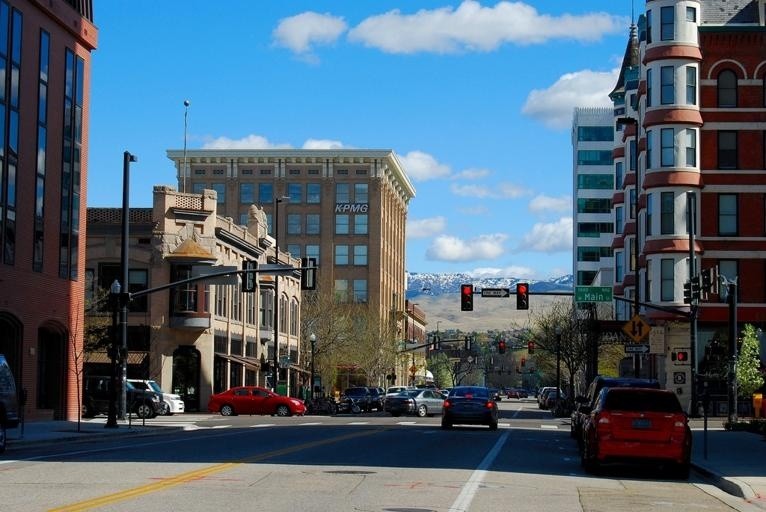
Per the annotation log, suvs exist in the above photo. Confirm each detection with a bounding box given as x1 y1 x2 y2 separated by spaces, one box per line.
82 375 185 419
0 353 19 453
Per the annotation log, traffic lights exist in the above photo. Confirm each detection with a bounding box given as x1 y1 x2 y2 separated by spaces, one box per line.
528 340 534 354
671 352 688 362
499 340 505 354
461 284 473 311
498 358 534 376
684 269 709 304
517 283 528 310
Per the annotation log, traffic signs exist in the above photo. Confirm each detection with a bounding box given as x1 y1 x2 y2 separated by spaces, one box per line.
574 286 613 302
625 344 650 354
482 288 510 297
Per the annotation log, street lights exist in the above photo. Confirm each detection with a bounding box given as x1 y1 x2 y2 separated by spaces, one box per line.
104 279 121 428
309 333 316 400
618 116 641 314
555 325 563 402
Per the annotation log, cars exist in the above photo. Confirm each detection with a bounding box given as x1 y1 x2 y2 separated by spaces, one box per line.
305 385 453 417
571 376 692 470
442 386 499 431
532 386 568 410
208 386 307 416
505 388 528 399
